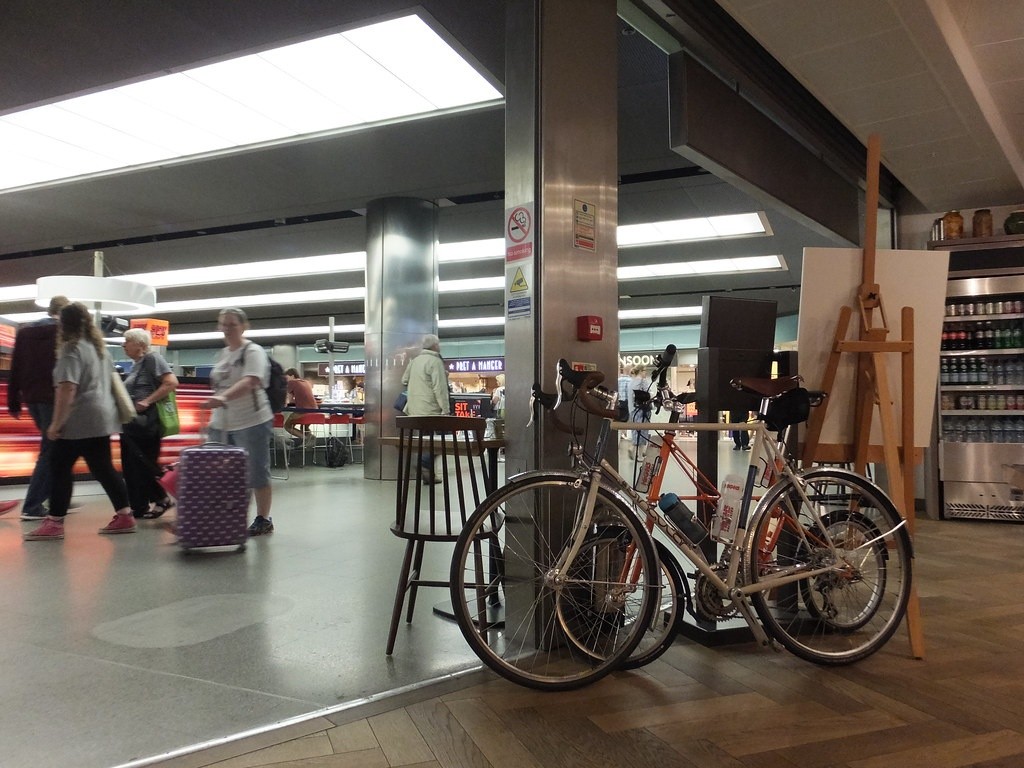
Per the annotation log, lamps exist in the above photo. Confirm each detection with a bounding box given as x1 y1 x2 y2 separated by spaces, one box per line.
313 339 349 353
99 314 129 334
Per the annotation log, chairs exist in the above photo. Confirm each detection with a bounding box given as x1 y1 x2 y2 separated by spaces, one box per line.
385 414 505 655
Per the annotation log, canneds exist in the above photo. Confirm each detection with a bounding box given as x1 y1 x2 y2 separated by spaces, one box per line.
945 301 1023 315
941 394 1024 410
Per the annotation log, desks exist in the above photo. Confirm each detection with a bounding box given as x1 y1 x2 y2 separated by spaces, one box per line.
377 434 505 628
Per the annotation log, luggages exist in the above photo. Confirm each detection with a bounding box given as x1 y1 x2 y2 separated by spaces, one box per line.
118 422 180 500
177 402 250 555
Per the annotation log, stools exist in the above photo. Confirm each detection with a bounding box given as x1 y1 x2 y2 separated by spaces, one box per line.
268 412 366 479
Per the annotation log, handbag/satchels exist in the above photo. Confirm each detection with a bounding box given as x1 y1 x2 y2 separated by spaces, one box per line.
111 371 137 425
394 390 407 410
155 391 180 437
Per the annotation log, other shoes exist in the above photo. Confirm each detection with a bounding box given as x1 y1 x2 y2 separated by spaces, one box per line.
637 455 645 462
742 444 751 450
306 435 316 451
293 441 308 450
0 500 19 515
423 475 442 485
628 447 635 460
733 443 741 450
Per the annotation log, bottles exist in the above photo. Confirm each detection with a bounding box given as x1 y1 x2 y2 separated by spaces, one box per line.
634 434 663 493
940 320 1024 444
658 493 706 544
713 473 744 541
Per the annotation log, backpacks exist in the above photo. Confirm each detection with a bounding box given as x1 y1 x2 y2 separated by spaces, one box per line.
325 436 349 468
240 341 287 413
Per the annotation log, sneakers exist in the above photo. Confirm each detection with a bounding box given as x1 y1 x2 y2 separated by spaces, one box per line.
98 513 137 534
20 506 49 520
22 518 65 540
247 516 274 537
67 504 82 513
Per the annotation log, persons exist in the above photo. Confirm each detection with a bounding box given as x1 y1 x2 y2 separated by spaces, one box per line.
284 368 317 449
24 303 137 541
492 373 505 454
208 308 274 536
619 362 650 462
729 411 751 450
402 335 451 484
121 329 178 519
6 296 82 519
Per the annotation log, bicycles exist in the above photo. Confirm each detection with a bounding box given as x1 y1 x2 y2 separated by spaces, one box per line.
550 344 890 670
449 358 916 692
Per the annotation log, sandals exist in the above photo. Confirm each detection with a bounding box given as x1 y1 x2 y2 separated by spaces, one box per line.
142 498 173 520
113 509 140 520
